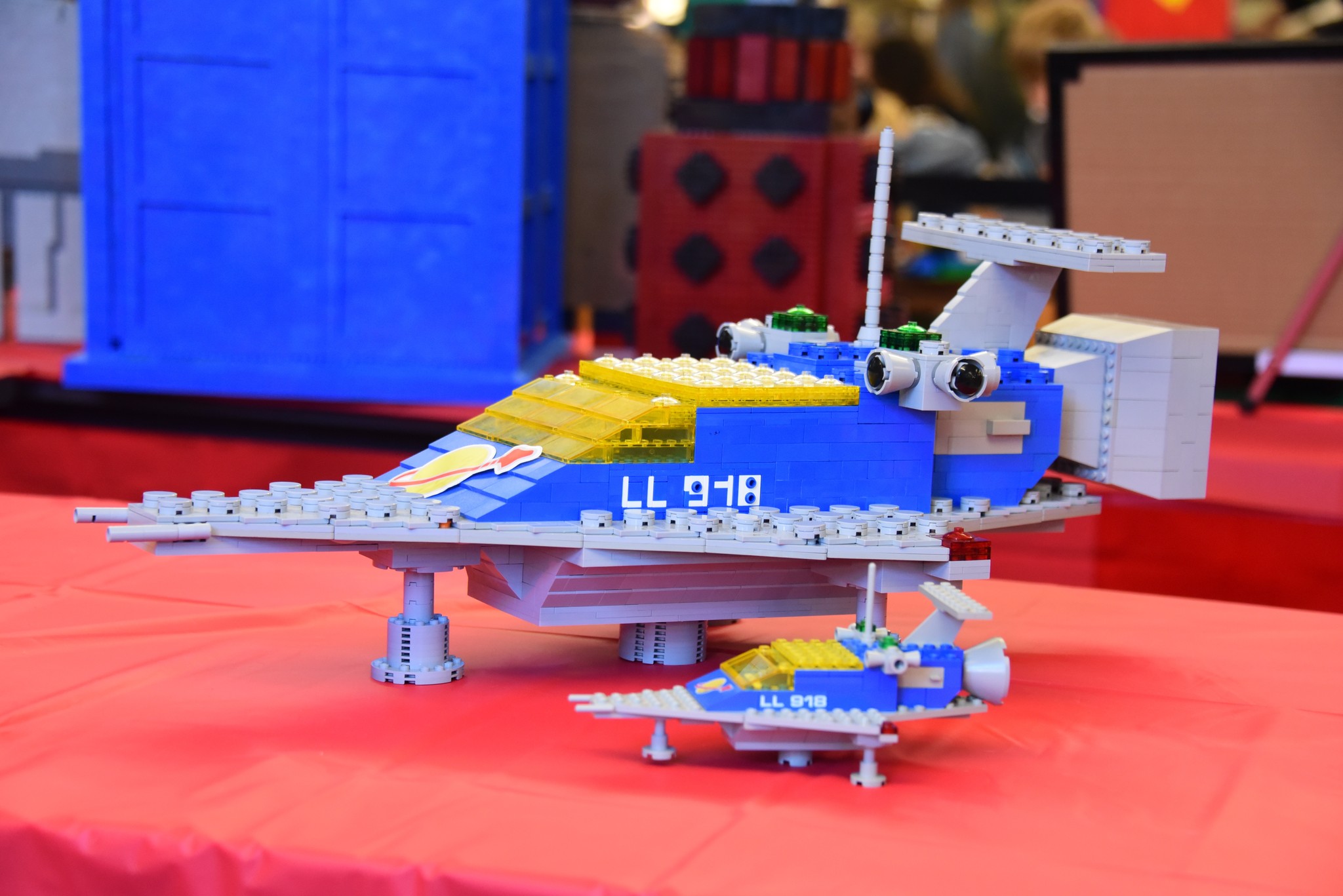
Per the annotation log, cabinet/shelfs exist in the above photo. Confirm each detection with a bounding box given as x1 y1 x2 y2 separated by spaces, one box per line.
64 0 567 407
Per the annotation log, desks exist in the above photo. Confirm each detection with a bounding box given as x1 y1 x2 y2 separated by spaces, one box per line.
0 493 1343 896
0 287 1343 616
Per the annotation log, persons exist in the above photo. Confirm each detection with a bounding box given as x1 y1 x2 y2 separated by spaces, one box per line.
994 0 1113 173
852 31 982 174
